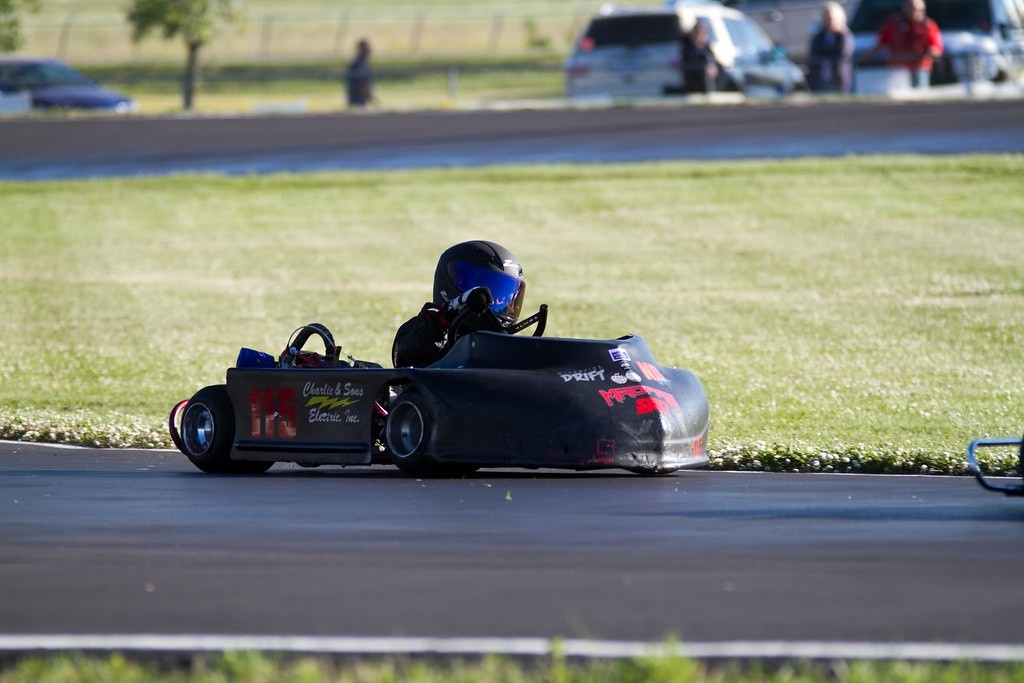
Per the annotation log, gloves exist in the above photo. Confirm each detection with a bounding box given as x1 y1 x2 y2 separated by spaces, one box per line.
438 286 494 326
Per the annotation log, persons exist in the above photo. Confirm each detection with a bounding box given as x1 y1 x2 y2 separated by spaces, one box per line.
684 25 717 96
808 2 854 94
883 0 943 88
392 240 526 369
343 39 374 111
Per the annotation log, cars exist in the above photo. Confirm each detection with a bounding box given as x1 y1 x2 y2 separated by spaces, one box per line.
810 0 1024 95
565 0 811 99
0 58 132 115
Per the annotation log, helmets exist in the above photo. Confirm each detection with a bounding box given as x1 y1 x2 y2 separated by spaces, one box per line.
432 239 524 327
